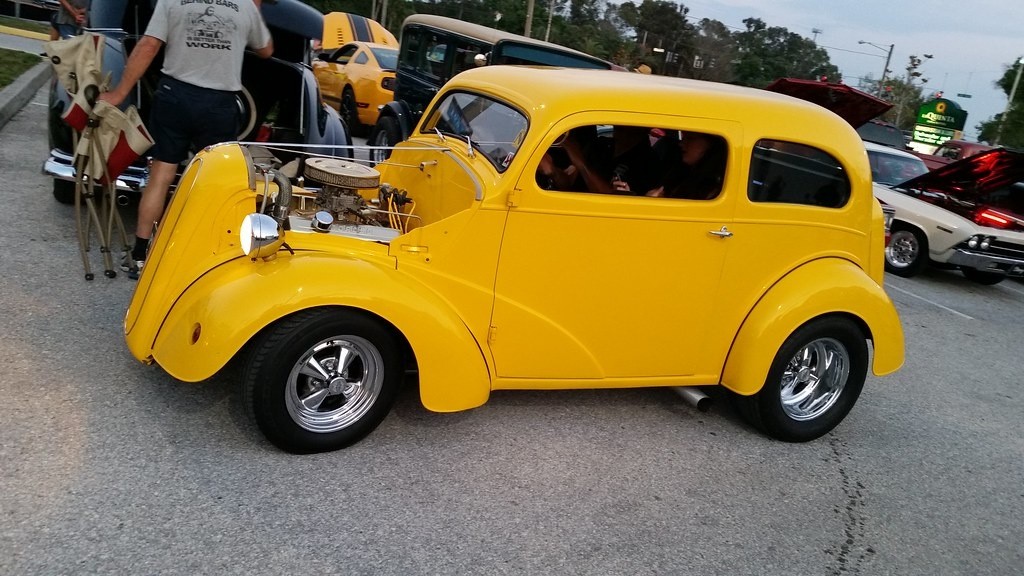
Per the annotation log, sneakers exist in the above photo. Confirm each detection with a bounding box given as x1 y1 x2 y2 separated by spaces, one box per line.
121 254 145 275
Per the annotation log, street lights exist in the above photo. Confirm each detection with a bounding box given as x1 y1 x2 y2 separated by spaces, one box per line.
858 39 894 99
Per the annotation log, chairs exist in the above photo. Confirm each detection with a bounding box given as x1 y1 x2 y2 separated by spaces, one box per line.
45 35 156 281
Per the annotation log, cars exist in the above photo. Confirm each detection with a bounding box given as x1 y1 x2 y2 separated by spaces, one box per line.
766 75 1024 285
41 5 628 206
121 64 904 456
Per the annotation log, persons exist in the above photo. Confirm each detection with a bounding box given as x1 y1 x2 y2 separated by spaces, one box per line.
95 0 273 273
541 124 719 199
635 64 652 74
40 0 89 57
